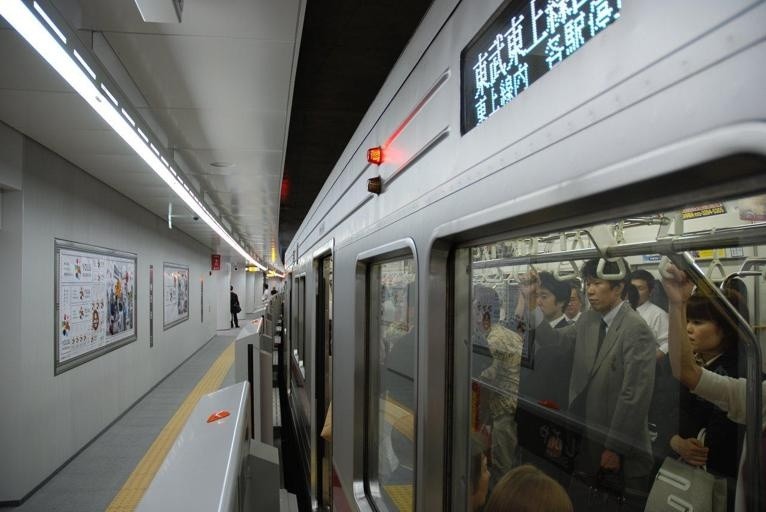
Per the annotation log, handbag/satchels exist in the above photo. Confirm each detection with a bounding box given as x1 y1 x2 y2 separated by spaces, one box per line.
644 457 727 512
234 304 241 313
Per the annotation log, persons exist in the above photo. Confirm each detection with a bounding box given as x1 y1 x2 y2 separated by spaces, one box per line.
230 285 241 328
261 283 269 301
271 286 278 299
379 255 765 512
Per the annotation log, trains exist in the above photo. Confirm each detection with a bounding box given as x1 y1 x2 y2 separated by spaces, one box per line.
275 2 766 512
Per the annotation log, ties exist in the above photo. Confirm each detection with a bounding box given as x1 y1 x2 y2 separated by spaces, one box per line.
594 319 607 365
